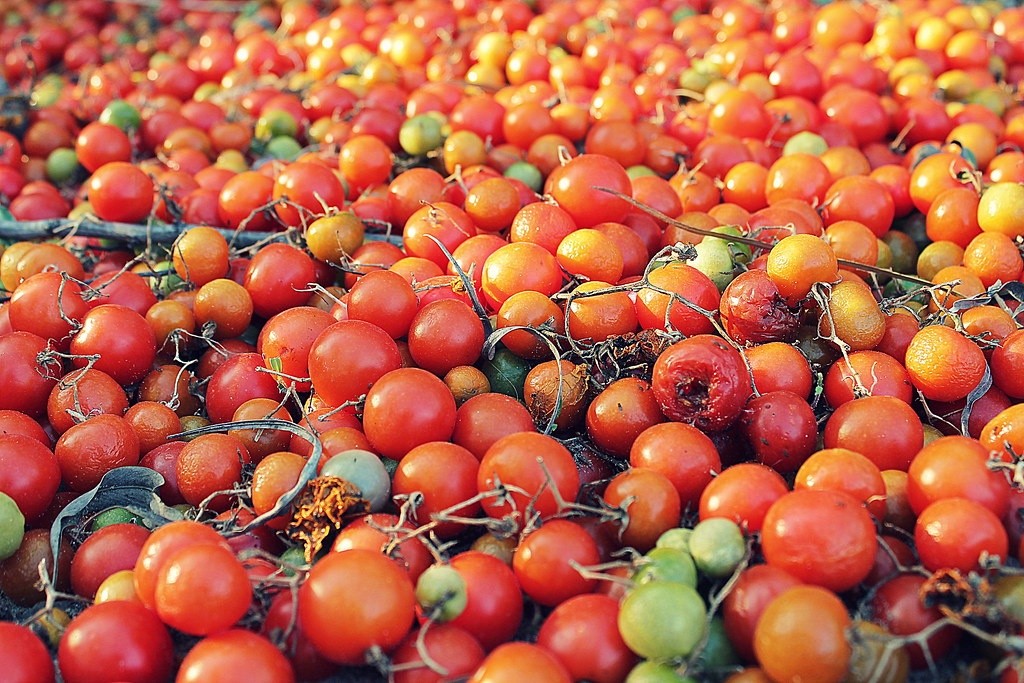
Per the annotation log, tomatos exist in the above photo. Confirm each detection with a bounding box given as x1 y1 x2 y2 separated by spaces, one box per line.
0 0 1024 683
